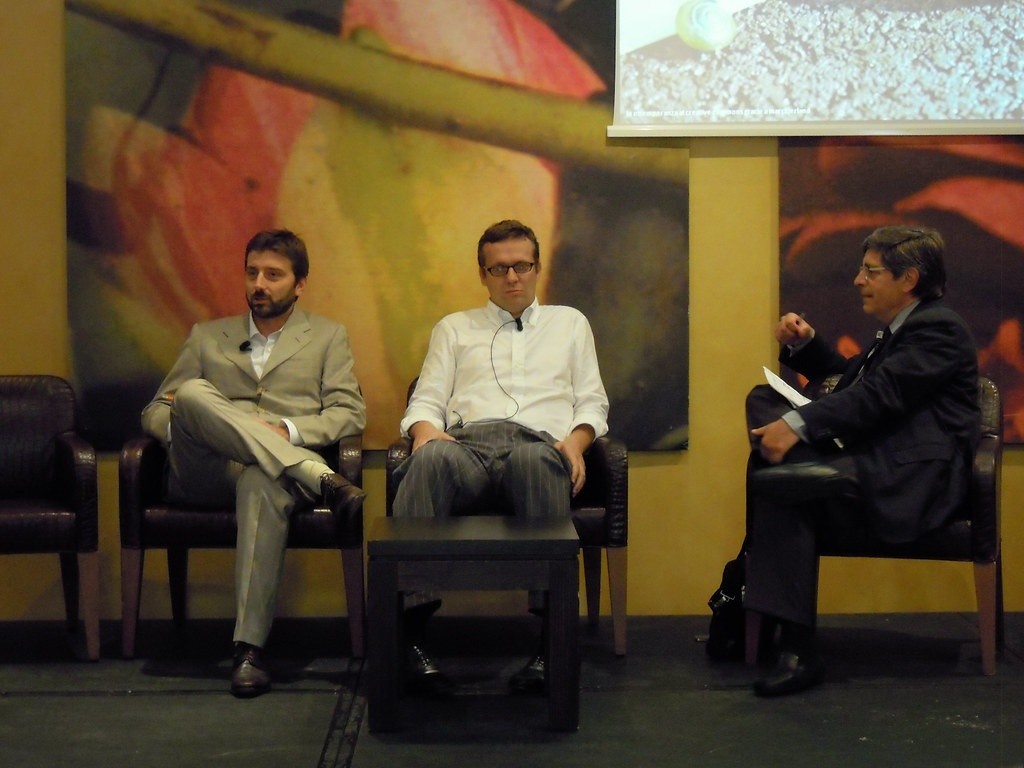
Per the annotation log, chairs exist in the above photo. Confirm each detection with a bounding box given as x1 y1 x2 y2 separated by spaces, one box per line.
0 375 100 663
122 363 364 663
386 373 629 656
747 372 1002 680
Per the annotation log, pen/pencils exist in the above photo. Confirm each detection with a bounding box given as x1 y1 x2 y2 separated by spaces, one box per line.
795 311 806 325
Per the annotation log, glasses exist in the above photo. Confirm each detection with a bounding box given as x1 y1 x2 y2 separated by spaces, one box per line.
858 265 889 282
482 261 537 277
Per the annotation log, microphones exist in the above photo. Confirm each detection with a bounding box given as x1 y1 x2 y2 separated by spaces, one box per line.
239 340 254 353
514 316 523 332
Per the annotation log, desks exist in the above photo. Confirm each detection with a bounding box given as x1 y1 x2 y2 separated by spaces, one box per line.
361 513 582 735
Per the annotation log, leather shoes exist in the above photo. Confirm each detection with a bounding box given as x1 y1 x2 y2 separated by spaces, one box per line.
319 472 365 523
752 647 825 696
755 463 860 495
231 641 272 699
399 641 438 682
509 645 550 690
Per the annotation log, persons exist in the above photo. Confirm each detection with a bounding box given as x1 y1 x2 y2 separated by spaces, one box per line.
142 227 366 694
393 219 610 693
750 227 982 701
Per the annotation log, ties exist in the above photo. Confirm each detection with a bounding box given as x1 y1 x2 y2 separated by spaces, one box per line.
858 325 892 376
